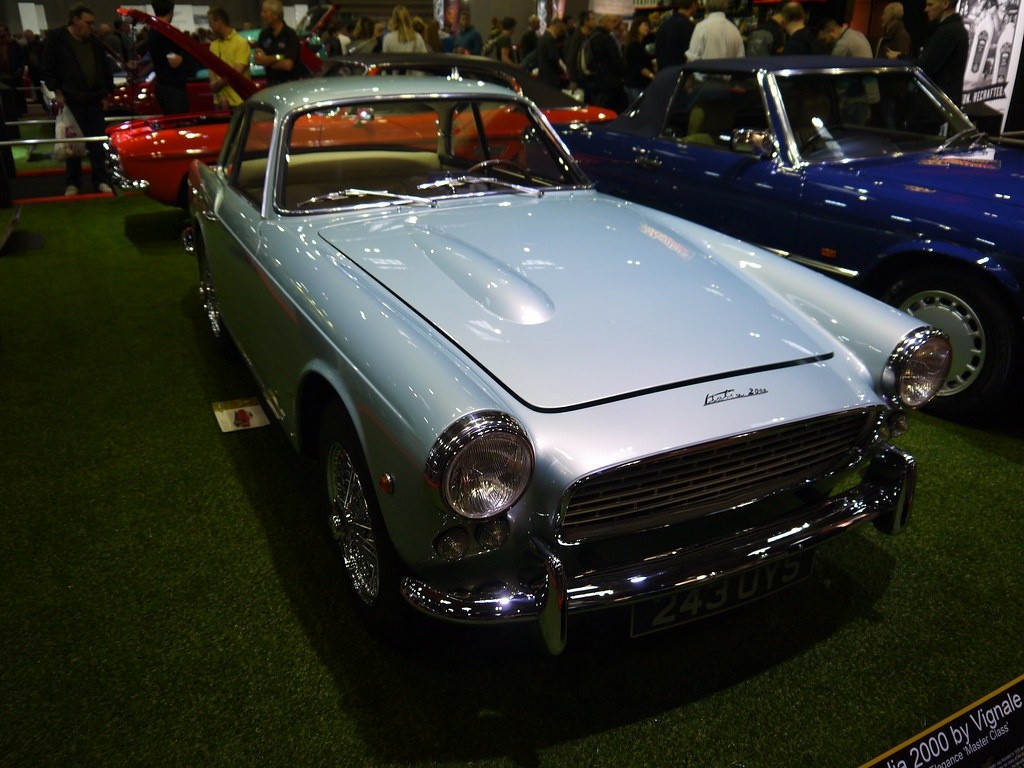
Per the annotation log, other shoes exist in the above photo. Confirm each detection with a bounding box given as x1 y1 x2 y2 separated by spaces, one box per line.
98 181 112 193
64 184 78 196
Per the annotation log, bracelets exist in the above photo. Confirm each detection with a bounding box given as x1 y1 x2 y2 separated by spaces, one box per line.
650 72 653 79
276 54 280 62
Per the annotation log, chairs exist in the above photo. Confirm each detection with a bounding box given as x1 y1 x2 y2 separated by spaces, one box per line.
789 95 846 158
681 98 737 152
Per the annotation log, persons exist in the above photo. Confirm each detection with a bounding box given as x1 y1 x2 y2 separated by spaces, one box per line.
1 0 968 206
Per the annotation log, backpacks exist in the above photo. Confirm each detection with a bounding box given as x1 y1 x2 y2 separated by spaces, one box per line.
576 32 605 86
747 28 773 57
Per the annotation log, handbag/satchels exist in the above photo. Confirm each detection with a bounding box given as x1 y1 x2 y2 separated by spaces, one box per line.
561 87 584 104
53 103 87 160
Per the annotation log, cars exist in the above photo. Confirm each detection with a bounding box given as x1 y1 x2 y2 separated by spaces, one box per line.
523 53 1024 426
100 51 618 226
187 75 951 641
100 0 337 124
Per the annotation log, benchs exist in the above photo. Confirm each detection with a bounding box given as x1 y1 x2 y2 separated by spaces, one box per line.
234 151 448 213
